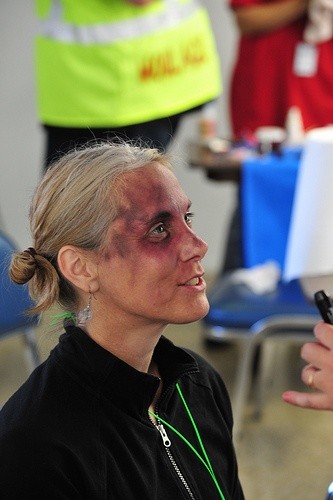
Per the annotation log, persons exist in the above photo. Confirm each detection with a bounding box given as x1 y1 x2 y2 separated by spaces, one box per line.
0 144 247 500
33 1 221 176
282 317 333 499
204 0 333 408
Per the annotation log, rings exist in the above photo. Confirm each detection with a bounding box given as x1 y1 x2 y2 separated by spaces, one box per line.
307 368 315 388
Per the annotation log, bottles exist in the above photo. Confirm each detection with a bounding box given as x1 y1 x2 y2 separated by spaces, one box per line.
293 14 319 77
200 99 219 148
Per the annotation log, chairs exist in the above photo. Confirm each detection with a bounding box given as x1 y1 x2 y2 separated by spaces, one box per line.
200 147 333 438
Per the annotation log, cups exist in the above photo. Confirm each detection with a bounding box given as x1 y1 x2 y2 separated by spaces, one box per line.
256 126 287 157
